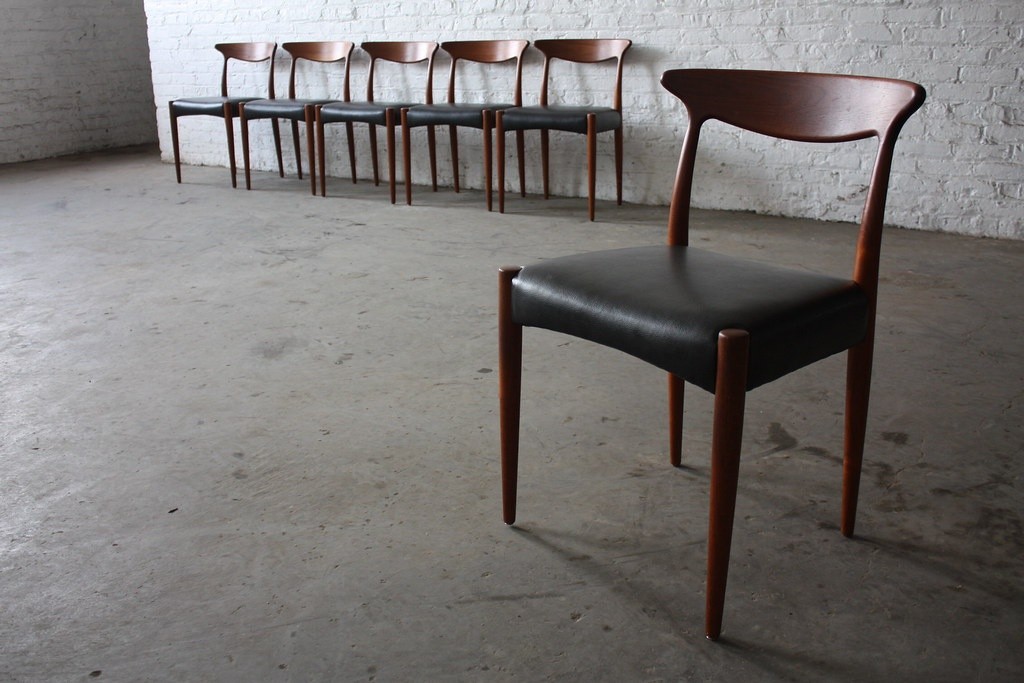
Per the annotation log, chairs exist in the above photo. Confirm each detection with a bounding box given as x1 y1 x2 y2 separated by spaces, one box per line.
497 67 927 641
238 41 357 196
168 42 284 189
314 42 438 205
400 40 530 211
496 38 632 222
